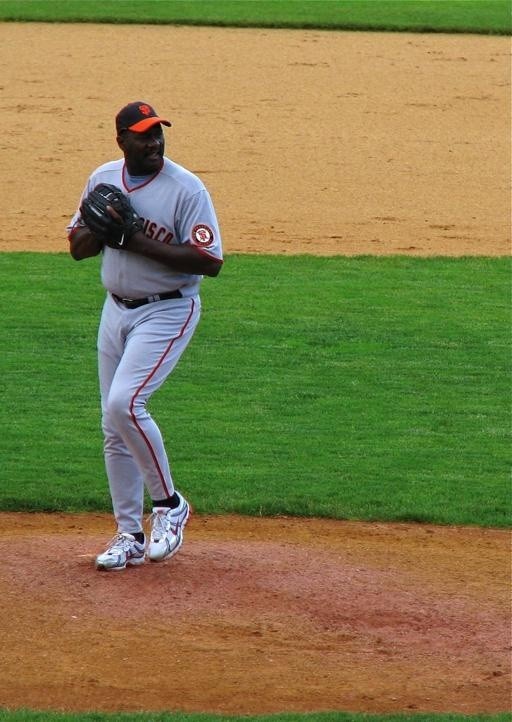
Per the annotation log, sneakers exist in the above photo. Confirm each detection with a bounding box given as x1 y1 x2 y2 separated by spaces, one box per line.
95 532 146 572
146 490 193 562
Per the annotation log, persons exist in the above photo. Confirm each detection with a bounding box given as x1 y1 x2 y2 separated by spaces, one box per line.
67 101 224 573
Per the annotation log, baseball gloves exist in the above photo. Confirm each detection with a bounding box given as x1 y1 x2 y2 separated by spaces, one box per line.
80 183 144 251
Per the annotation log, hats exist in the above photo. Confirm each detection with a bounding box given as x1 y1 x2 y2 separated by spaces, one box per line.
116 102 171 136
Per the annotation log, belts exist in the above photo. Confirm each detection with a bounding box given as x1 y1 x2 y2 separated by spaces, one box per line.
112 289 182 309
